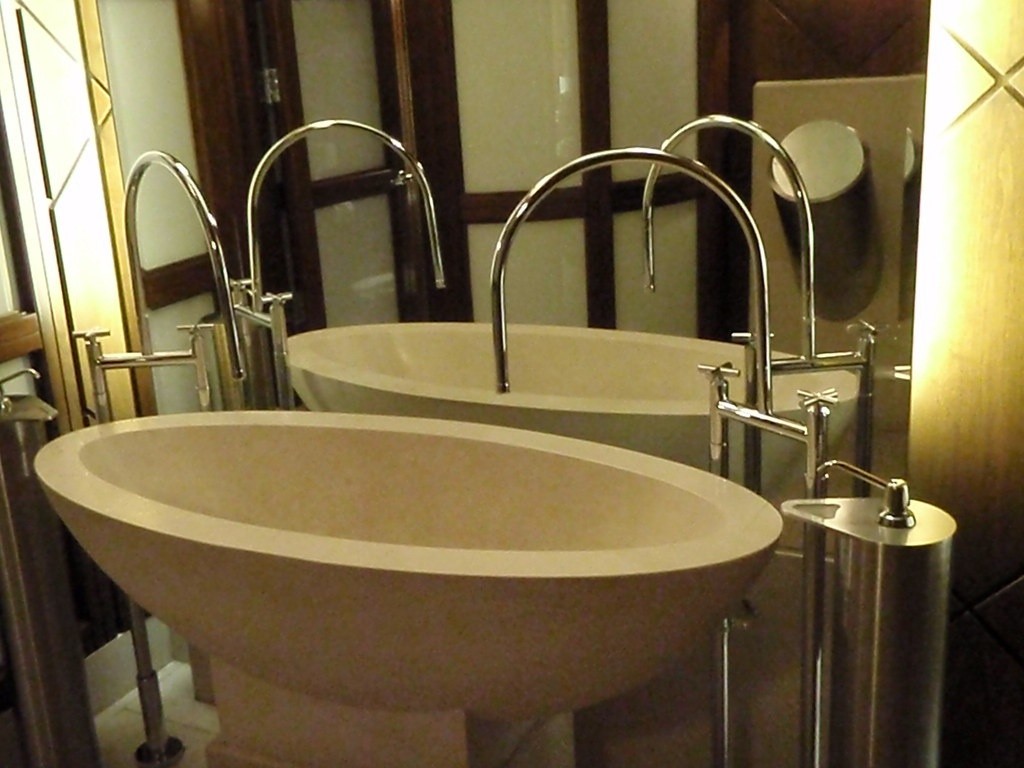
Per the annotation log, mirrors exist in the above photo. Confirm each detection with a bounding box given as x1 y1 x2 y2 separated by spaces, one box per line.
93 2 931 707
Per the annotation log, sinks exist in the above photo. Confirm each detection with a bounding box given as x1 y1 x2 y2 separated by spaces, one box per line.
276 321 859 491
32 410 785 711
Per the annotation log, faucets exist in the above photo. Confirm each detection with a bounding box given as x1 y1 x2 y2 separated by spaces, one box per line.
122 149 245 382
641 120 817 355
247 120 446 309
488 148 772 414
